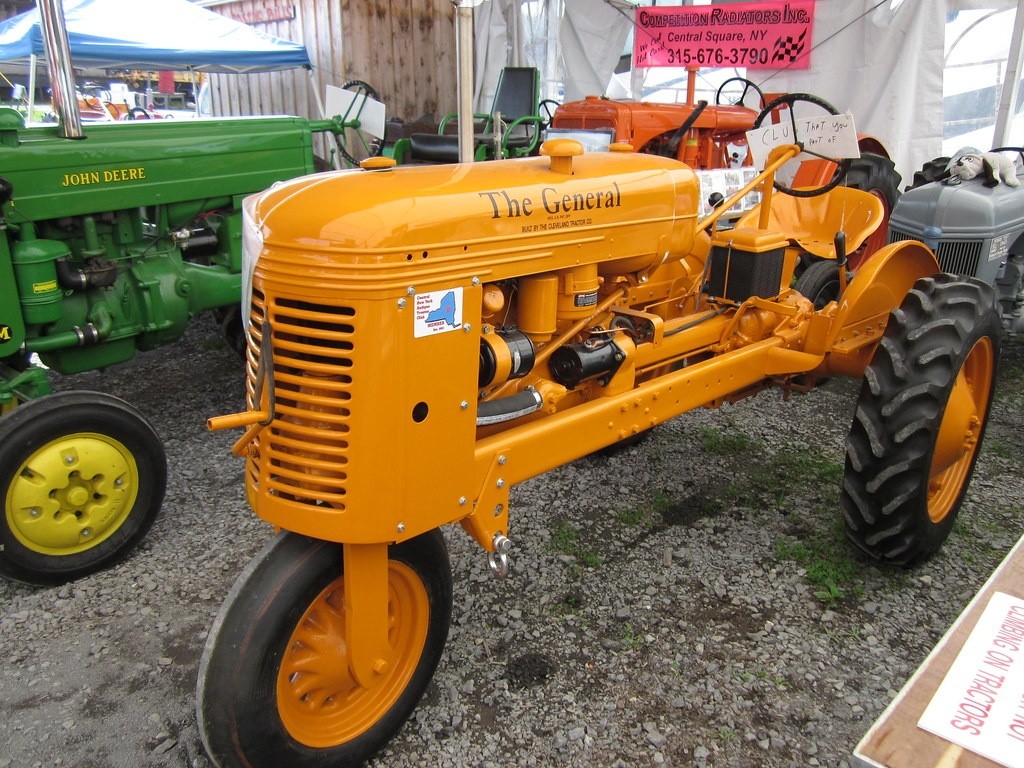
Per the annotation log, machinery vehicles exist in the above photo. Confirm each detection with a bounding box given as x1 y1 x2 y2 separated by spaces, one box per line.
0 0 551 591
179 71 1003 768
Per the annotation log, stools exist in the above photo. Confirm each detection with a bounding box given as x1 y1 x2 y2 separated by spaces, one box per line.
733 184 885 262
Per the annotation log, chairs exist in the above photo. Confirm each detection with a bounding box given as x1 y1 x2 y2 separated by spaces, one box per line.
409 63 540 166
